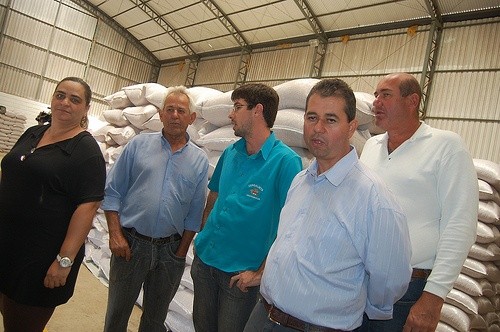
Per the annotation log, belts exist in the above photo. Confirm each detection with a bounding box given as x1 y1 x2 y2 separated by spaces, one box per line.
412 270 431 278
262 298 335 332
123 227 182 244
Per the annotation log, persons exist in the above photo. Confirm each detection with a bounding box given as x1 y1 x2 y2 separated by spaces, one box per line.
189 83 303 332
99 85 209 332
243 78 414 332
358 71 479 332
0 76 107 332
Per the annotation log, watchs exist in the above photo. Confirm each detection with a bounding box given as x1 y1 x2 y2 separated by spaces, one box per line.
57 254 73 268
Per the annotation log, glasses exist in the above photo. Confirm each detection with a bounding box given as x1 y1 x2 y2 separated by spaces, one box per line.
233 104 254 112
